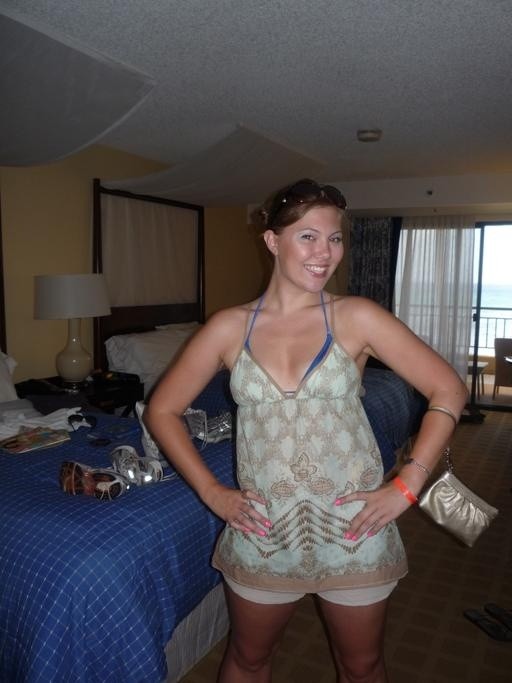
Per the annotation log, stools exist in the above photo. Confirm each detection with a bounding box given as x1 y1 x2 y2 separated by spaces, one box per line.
465 359 492 401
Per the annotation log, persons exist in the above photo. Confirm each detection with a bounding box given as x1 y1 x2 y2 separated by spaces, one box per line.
141 178 470 683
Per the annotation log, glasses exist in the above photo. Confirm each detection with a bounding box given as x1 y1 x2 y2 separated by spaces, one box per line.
278 178 347 210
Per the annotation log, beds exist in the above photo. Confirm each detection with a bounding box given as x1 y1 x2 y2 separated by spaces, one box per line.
0 387 243 683
84 308 423 494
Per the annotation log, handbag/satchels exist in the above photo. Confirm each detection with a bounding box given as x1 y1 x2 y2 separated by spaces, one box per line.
420 472 499 548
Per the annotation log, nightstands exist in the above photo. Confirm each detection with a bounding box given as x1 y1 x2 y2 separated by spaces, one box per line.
11 368 149 427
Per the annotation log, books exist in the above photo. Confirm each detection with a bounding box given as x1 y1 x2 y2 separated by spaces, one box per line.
0 425 71 454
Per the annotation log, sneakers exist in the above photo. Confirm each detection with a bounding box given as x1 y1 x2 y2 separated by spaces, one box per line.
60 402 237 500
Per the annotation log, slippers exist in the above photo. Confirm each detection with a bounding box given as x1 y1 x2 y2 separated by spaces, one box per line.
463 603 512 640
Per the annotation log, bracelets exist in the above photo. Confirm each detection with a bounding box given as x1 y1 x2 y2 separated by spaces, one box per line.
394 476 417 505
404 458 430 480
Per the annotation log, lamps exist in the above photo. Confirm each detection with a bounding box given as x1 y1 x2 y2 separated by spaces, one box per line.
30 267 118 387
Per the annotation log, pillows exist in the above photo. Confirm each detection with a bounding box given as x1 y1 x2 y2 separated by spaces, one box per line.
0 346 21 405
101 316 215 406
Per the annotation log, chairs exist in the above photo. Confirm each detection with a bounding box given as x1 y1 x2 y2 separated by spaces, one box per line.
488 336 512 403
